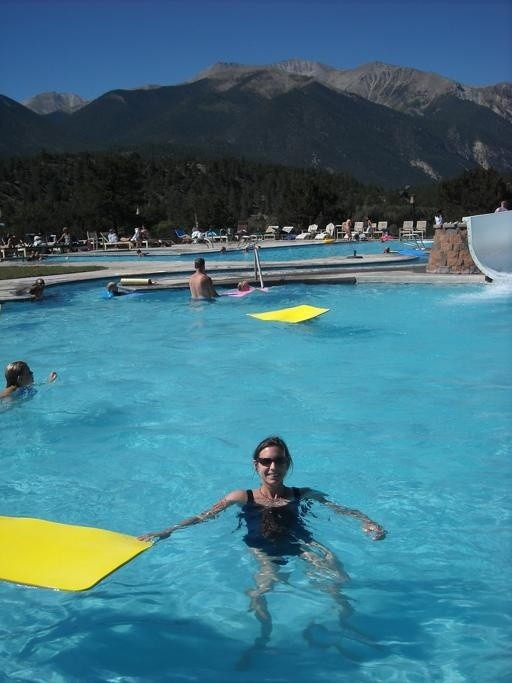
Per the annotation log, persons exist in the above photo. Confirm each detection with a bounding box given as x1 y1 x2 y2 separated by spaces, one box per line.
367 215 373 227
139 431 387 621
207 225 217 241
0 230 58 260
188 257 220 298
494 200 508 212
384 247 390 253
434 209 442 223
107 223 151 248
237 279 250 291
107 281 119 293
344 216 352 237
137 248 149 257
10 276 46 300
58 226 72 245
191 226 205 242
0 360 59 400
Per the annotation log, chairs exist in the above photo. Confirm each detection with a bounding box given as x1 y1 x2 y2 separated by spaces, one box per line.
234 219 249 239
398 219 427 241
86 228 154 248
174 226 201 242
264 219 389 240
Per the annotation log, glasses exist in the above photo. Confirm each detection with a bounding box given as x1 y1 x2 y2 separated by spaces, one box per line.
257 457 288 466
